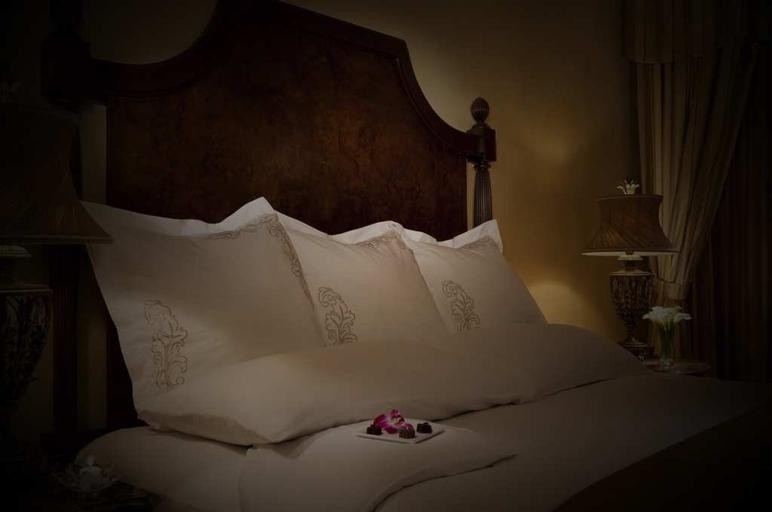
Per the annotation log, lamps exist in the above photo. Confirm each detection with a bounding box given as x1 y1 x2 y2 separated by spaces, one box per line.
580 180 680 361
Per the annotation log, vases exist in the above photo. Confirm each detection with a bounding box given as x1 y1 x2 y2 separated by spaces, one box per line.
653 323 676 372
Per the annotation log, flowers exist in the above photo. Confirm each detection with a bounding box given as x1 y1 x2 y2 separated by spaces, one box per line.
642 302 692 359
372 408 415 435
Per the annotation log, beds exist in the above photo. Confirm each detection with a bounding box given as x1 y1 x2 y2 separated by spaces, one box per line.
61 1 769 510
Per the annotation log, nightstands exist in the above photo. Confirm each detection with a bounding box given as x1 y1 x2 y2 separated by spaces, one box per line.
643 360 711 376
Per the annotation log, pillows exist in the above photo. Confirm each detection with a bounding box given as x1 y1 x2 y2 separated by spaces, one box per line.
390 215 551 338
270 205 450 349
137 343 531 443
452 323 657 407
71 194 326 433
